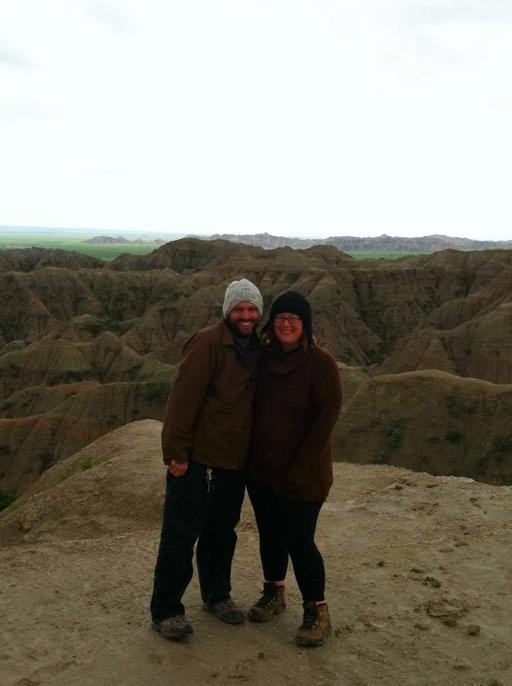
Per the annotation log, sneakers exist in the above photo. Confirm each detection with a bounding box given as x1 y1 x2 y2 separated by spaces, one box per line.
296 602 333 646
202 597 246 624
151 615 193 640
248 582 287 623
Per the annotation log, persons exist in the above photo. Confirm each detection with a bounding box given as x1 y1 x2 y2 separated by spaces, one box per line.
243 289 344 651
148 276 264 642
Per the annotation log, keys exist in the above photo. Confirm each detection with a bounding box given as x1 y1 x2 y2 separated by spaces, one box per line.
205 466 216 492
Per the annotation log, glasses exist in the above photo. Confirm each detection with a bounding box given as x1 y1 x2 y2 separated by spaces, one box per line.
274 316 301 325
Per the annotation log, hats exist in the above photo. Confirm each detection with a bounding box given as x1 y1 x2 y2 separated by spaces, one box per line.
270 291 311 328
222 278 264 322
